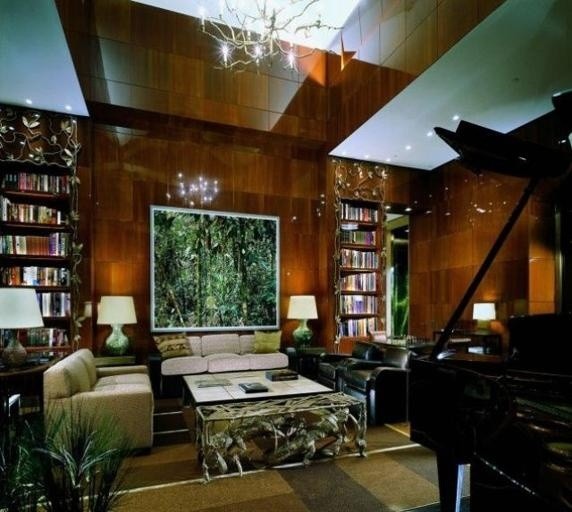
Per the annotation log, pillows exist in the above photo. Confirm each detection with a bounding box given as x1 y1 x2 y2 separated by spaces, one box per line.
152 331 195 360
252 329 283 354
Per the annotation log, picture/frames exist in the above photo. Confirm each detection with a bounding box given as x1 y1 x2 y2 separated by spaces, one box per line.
148 202 280 333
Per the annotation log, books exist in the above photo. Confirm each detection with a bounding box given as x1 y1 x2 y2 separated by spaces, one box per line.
238 380 269 394
337 199 380 339
265 369 299 381
2 171 71 358
195 378 234 388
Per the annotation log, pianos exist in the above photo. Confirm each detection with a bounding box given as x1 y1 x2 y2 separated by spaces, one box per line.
407 117 569 512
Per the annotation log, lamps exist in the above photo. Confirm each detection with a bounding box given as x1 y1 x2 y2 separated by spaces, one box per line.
95 294 139 356
191 0 348 80
285 295 318 352
470 302 496 336
0 287 45 370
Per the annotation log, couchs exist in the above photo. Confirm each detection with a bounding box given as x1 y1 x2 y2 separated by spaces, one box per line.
160 331 288 400
42 348 156 457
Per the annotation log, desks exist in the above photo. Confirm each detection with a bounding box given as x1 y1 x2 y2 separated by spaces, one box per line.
0 363 52 434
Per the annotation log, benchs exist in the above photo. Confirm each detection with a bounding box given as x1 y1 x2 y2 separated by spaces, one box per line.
194 391 366 481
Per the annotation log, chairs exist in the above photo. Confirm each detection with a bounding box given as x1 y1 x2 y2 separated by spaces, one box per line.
312 342 418 427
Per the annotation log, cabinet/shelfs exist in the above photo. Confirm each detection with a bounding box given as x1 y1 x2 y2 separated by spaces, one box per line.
319 197 386 356
0 157 80 367
